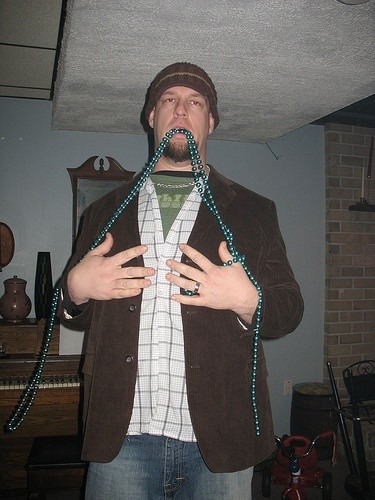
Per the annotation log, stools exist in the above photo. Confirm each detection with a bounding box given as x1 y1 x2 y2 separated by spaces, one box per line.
24 437 89 500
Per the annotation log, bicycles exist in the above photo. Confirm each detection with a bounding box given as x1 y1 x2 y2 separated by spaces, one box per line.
262 431 333 500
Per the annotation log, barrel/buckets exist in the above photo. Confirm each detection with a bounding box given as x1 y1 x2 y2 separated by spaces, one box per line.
290 383 338 460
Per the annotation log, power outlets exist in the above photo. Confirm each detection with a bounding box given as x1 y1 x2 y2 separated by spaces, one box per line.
282 379 293 397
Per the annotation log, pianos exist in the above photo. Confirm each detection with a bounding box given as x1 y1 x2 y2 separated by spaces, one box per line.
0 355 80 436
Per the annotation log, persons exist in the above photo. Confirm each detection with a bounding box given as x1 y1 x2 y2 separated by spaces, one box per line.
52 62 306 500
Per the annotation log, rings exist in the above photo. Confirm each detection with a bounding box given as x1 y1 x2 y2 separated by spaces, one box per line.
194 282 200 294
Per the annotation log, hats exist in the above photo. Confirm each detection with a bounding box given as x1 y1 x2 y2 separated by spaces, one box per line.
143 61 220 130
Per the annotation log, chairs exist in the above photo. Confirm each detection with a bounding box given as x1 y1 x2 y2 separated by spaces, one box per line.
340 360 375 500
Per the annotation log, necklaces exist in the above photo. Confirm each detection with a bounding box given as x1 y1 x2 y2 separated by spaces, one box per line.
149 174 197 189
4 128 265 435
